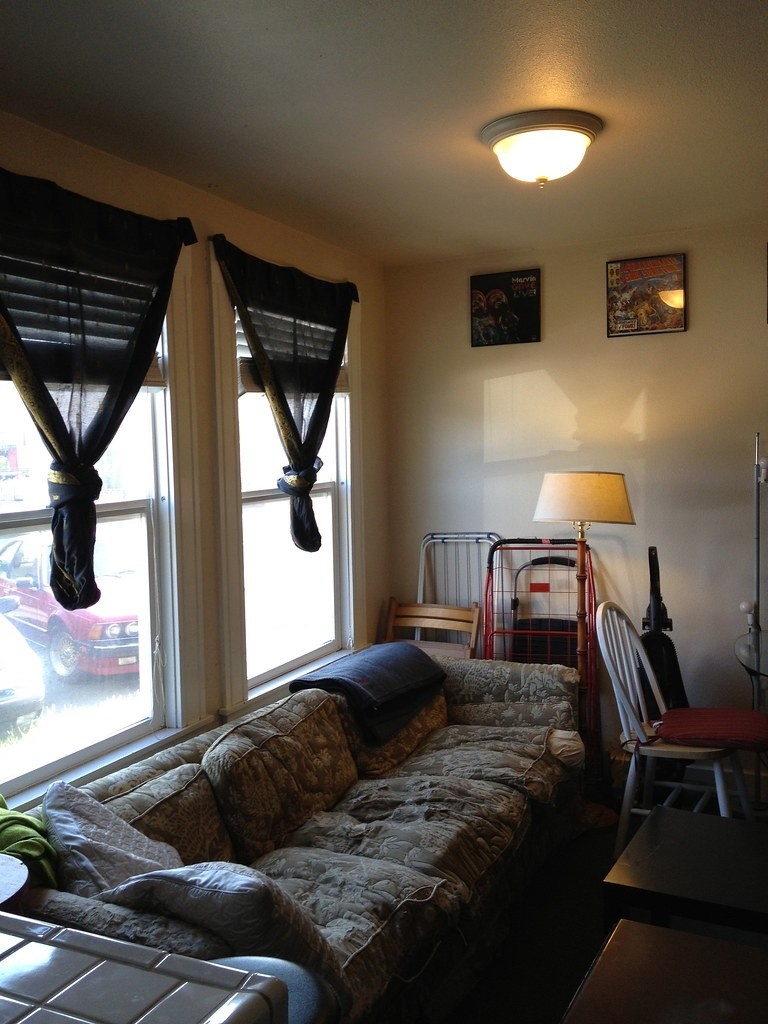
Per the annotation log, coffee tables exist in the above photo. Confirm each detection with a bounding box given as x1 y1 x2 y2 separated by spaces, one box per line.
556 916 767 1024
603 804 768 937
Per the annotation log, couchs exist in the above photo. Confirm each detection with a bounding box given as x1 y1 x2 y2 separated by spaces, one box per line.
0 657 586 1024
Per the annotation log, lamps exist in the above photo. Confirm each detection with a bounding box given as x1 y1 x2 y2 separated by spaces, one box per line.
477 108 604 189
534 470 637 744
738 431 767 678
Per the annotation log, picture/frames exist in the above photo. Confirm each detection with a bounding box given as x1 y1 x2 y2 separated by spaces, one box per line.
605 252 688 339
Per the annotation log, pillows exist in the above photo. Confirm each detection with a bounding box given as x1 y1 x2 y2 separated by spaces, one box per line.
99 763 238 865
90 861 354 1024
326 685 449 776
42 782 184 898
202 688 358 866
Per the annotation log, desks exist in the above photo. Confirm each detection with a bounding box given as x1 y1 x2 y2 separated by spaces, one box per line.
733 629 767 819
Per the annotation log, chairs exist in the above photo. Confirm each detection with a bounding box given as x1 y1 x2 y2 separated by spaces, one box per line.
595 601 768 861
377 595 482 660
